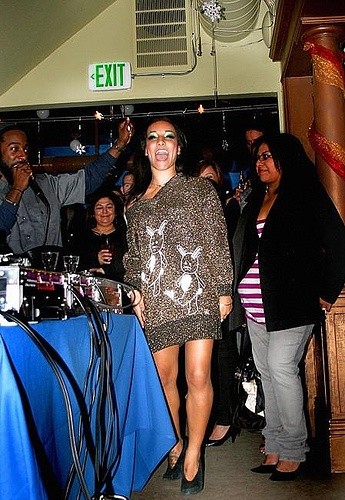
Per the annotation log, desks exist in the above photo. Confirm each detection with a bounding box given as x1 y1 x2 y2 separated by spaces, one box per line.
0 313 179 500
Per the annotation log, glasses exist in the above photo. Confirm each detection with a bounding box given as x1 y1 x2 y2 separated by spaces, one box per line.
255 153 272 162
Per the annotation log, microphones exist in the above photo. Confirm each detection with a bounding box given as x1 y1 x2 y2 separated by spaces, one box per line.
28 174 50 207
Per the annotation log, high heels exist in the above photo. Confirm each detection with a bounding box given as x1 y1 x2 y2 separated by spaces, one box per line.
202 420 238 446
163 438 188 481
180 445 203 495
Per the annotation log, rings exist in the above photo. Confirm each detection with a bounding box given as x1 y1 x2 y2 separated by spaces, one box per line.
12 163 18 168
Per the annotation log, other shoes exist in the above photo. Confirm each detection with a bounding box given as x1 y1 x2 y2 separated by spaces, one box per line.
251 464 277 474
269 463 301 481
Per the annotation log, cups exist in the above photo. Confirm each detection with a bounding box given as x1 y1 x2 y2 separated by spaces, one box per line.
101 237 114 264
36 110 49 118
121 105 134 115
70 140 82 152
63 255 79 273
41 252 59 271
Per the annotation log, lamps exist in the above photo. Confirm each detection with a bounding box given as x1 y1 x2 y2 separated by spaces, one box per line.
69 140 86 155
125 105 134 115
37 110 50 119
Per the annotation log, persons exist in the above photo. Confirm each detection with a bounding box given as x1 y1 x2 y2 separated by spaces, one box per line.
61 127 266 449
122 117 234 495
229 132 345 481
0 118 135 266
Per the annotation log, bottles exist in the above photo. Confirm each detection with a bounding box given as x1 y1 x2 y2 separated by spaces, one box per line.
238 170 246 192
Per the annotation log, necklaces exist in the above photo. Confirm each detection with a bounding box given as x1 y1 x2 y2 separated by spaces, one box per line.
151 183 166 187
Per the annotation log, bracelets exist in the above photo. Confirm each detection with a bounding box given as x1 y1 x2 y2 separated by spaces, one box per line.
10 188 23 194
218 298 233 306
111 143 124 153
